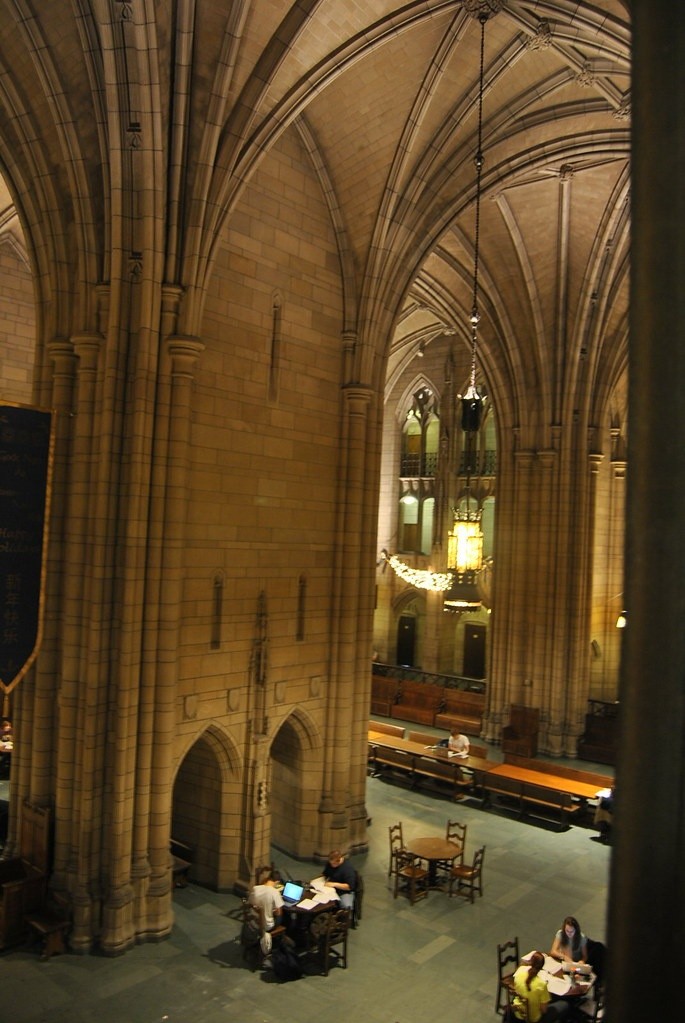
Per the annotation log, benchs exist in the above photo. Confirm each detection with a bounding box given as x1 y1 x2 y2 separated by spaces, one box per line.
367 674 485 739
473 769 581 830
504 753 617 790
367 745 415 787
412 757 473 802
169 837 195 889
408 731 487 759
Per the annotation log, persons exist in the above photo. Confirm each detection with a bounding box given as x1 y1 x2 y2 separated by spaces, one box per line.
509 916 588 1023
0 719 13 742
447 728 469 755
246 849 359 957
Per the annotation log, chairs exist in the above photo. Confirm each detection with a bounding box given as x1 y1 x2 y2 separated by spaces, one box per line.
387 822 422 877
435 820 467 883
240 866 364 977
21 880 72 962
393 847 430 907
495 937 606 1023
0 795 51 953
448 844 486 905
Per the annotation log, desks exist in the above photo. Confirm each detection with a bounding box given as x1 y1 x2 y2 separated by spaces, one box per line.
280 890 337 956
521 954 596 1003
367 731 613 826
405 837 463 893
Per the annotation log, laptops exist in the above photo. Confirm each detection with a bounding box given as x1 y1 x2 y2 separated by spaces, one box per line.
561 961 593 976
281 881 304 908
436 747 454 759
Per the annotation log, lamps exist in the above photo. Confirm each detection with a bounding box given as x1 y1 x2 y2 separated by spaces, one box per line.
392 0 507 590
